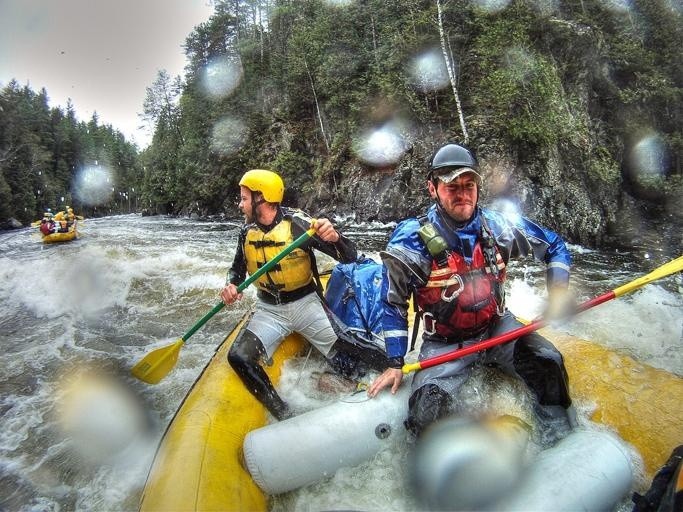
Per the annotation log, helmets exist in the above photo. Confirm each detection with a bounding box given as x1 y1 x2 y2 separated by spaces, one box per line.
239 167 285 205
427 141 480 173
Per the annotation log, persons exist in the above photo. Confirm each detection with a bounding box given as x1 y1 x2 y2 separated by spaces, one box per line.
218 167 369 422
365 144 579 452
39 203 76 237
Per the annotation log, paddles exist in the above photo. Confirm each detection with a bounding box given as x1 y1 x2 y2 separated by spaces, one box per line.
401 256 683 374
129 219 319 384
71 216 86 239
31 220 41 227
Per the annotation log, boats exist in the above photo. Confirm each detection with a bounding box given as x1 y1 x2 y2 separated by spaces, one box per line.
40 210 80 243
137 258 681 509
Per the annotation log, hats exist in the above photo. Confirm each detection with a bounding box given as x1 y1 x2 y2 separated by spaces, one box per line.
435 167 482 184
